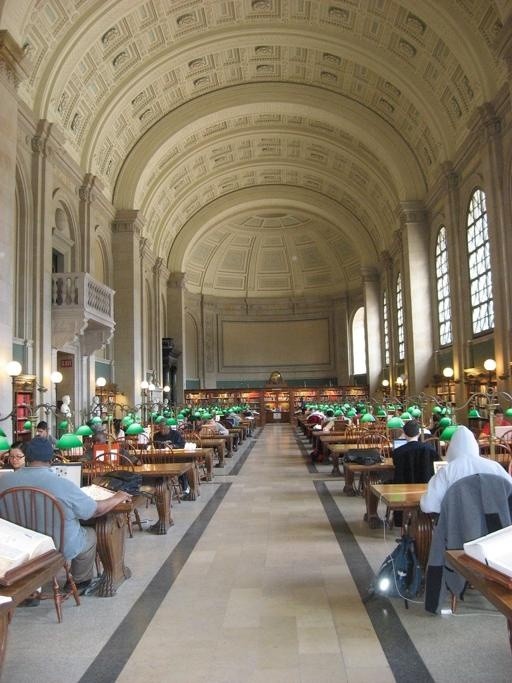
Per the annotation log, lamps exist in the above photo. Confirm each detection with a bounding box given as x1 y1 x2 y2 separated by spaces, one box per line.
297 358 512 442
0 361 243 452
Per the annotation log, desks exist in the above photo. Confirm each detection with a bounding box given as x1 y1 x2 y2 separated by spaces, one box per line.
0 494 145 598
368 480 512 594
0 549 66 676
444 546 512 649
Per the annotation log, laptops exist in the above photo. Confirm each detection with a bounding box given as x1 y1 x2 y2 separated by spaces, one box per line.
433 461 447 474
202 425 218 435
495 426 512 441
335 421 349 432
226 418 235 426
50 461 83 488
392 439 408 451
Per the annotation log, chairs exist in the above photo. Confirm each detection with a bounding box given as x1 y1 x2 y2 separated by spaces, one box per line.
296 413 512 527
0 423 251 534
1 487 82 627
441 473 509 612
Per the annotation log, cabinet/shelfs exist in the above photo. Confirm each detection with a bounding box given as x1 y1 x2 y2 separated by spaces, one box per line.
184 385 369 427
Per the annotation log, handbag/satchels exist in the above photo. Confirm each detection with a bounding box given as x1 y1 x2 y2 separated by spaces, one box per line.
343 447 383 465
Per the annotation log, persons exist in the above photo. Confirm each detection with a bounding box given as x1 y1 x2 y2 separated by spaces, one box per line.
61 394 72 418
104 403 255 491
151 398 159 411
163 398 170 409
0 434 134 607
0 440 28 471
91 395 100 416
300 399 511 529
35 420 60 445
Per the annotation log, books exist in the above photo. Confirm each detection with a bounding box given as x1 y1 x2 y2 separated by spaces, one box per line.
185 390 365 413
0 516 54 576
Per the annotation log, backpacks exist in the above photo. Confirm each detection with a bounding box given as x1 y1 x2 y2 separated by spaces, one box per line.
370 533 423 598
98 469 140 497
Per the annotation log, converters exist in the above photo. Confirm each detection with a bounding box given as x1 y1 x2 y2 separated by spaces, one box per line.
441 607 453 615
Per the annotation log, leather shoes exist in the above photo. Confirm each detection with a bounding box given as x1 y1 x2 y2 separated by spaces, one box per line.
19 591 40 607
63 579 91 593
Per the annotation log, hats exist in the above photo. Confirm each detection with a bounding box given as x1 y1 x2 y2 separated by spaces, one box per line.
26 435 52 461
